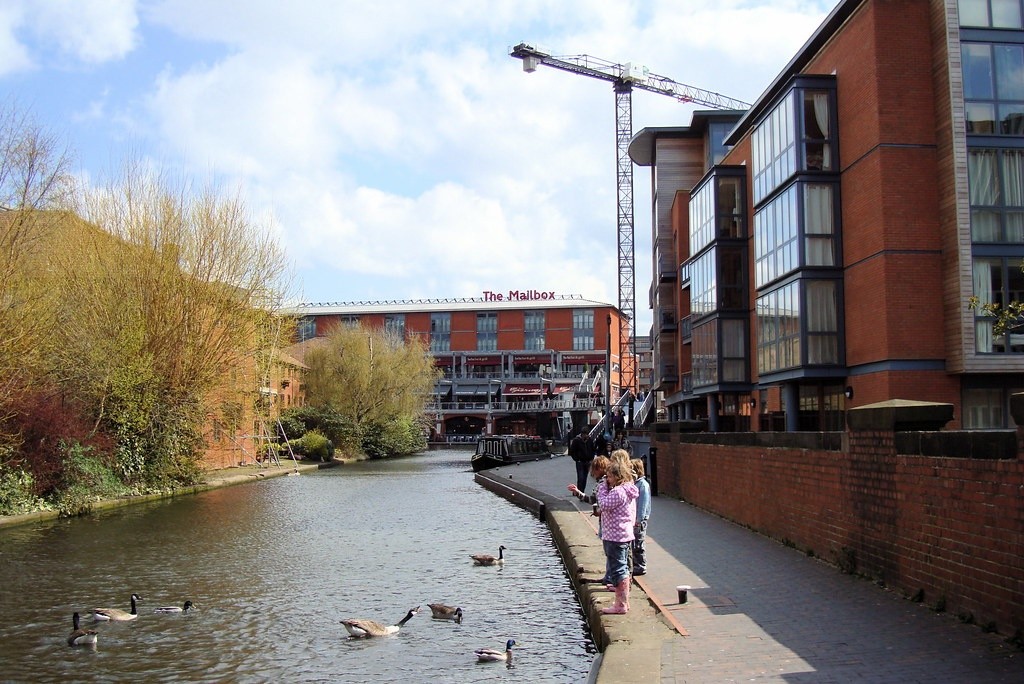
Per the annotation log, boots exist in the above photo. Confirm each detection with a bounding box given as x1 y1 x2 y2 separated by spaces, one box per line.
602 578 630 614
607 577 632 591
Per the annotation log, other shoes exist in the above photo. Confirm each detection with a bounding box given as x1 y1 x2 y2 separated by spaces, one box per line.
601 577 613 585
633 571 644 576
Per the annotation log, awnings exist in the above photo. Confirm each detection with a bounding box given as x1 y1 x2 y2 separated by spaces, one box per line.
562 354 606 364
553 384 601 395
433 358 460 366
476 385 500 395
454 386 478 395
432 385 451 395
467 357 501 366
502 384 550 396
515 356 551 364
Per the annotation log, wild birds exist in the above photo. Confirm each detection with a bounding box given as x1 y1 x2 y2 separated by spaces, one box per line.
153 600 197 614
66 611 99 644
86 594 142 620
468 545 508 566
339 606 421 638
426 604 463 624
473 639 516 661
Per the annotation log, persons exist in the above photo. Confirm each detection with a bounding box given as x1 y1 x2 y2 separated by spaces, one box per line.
593 366 599 377
644 388 649 398
594 432 633 457
637 391 641 400
609 406 626 434
567 449 650 614
546 396 550 406
569 428 594 493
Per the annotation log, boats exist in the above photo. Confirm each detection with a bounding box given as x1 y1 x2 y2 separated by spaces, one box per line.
470 433 555 473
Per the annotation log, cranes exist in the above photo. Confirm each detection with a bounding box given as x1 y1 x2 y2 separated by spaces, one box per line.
506 38 754 437
